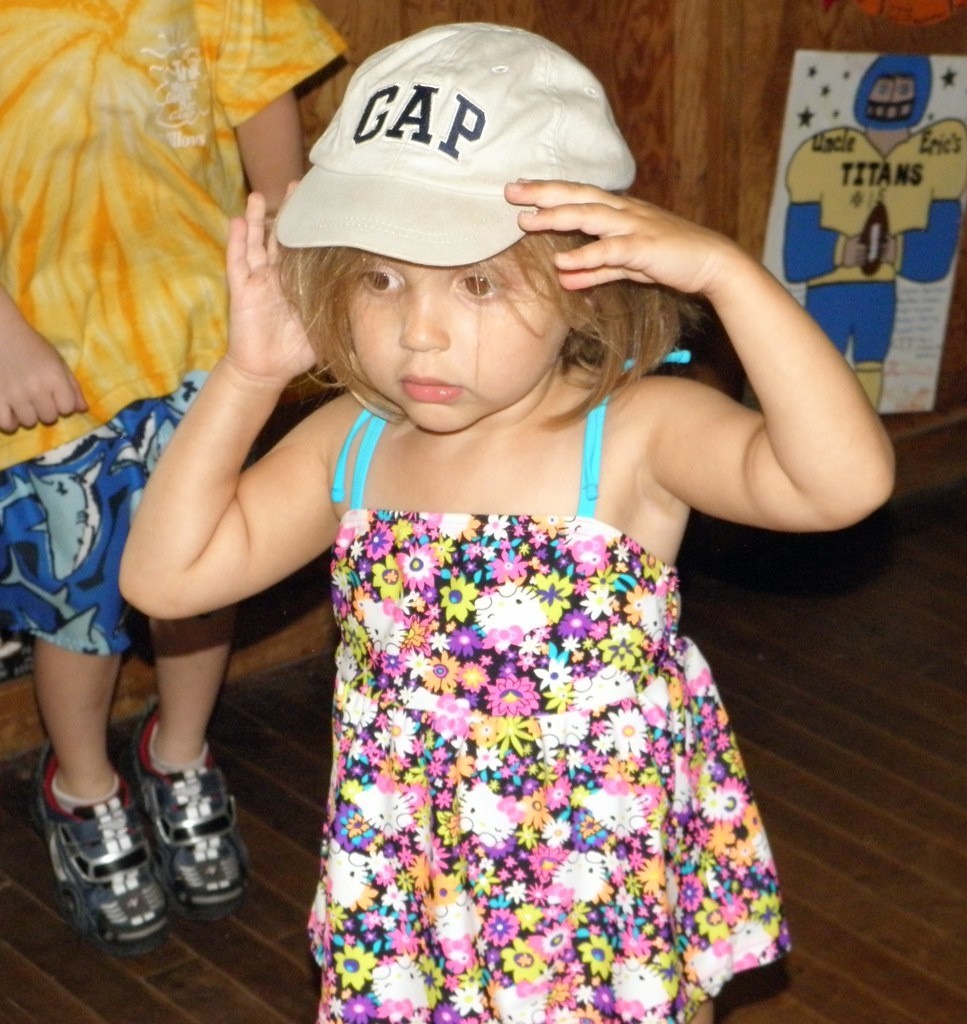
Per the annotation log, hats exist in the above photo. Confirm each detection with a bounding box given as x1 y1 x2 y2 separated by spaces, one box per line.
276 22 637 267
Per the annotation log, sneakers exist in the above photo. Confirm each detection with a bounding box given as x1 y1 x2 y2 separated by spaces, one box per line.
130 693 250 922
27 740 170 954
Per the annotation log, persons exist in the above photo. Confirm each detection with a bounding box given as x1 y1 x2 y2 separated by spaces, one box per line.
0 0 349 957
118 21 896 1024
